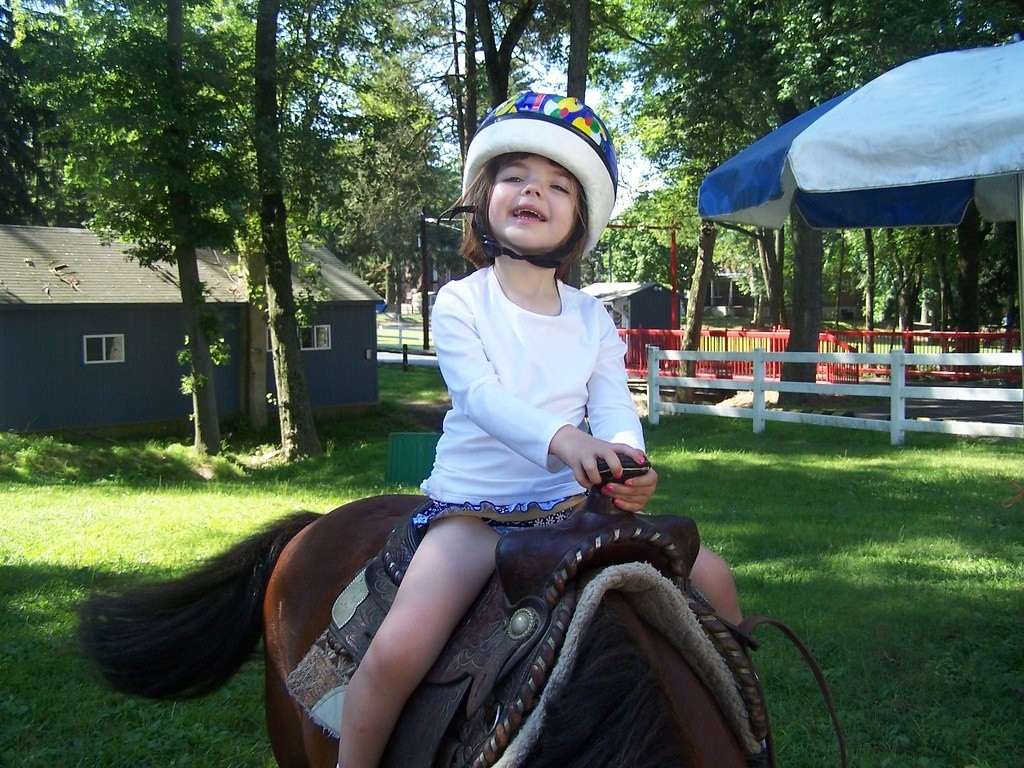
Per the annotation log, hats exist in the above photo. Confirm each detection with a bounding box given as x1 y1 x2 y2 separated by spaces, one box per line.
462 90 619 261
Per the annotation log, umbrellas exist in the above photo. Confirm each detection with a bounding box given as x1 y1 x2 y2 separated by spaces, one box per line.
697 38 1023 228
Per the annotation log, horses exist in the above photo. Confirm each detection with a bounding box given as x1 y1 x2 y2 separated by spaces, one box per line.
77 495 776 767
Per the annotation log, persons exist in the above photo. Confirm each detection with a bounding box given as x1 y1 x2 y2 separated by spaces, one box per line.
335 90 742 767
